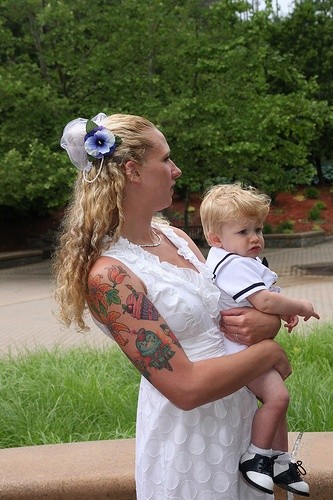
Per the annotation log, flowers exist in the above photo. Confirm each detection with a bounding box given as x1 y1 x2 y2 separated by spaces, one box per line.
59 112 125 183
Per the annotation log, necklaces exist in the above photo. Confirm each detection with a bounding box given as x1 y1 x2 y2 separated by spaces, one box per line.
136 228 161 247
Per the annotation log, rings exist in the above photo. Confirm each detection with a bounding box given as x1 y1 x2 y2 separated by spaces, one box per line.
235 333 240 341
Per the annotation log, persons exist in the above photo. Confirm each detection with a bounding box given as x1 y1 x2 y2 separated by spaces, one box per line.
53 112 293 500
201 182 321 497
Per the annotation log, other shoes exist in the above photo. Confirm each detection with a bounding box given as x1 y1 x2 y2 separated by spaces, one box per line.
274 460 311 497
239 450 274 494
